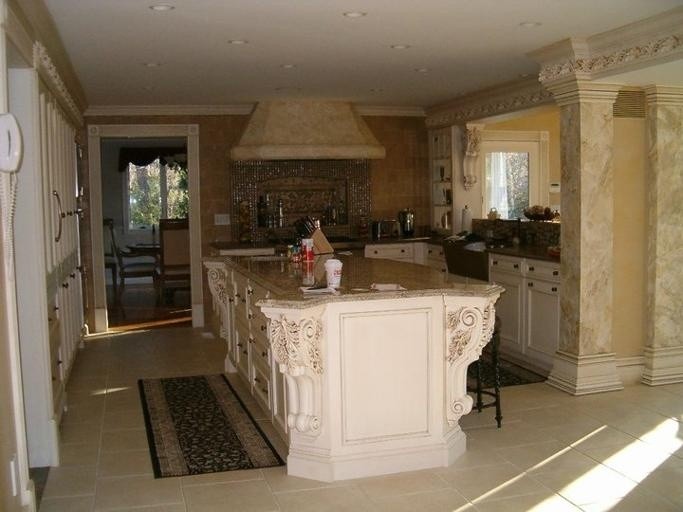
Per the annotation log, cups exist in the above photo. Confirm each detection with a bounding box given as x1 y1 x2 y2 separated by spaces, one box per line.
301 239 313 262
301 263 314 286
324 258 343 289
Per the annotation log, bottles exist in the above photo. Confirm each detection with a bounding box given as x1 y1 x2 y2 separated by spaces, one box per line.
400 207 415 237
286 245 300 262
286 264 300 281
257 193 283 228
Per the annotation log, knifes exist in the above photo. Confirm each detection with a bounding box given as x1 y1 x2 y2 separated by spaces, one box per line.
295 215 320 235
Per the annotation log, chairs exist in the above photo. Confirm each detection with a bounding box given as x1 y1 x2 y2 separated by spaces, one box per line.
103 218 190 309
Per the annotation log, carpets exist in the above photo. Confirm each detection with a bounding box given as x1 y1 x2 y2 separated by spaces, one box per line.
137 373 286 480
466 359 547 392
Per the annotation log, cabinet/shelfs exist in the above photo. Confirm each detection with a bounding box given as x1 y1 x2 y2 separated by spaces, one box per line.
364 242 560 378
8 70 83 467
224 268 288 441
433 157 452 207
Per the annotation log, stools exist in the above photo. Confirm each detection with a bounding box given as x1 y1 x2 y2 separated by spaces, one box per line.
466 315 503 429
